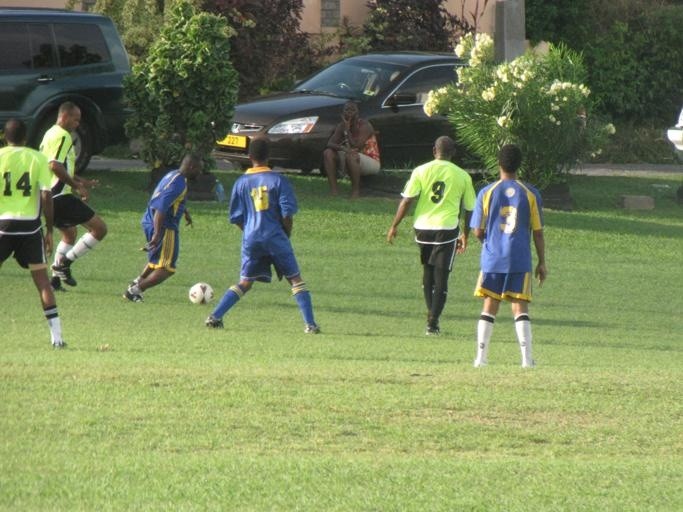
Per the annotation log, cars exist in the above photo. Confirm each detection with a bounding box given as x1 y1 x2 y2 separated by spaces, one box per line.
208 49 499 173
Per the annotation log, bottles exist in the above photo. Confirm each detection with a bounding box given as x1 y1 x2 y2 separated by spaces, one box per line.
215 179 227 202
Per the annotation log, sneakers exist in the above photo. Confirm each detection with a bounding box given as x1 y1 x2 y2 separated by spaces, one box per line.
51 277 65 289
52 340 66 348
426 325 439 336
125 280 143 303
51 254 76 286
206 316 224 328
304 323 319 334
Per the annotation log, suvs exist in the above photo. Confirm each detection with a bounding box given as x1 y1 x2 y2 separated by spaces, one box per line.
1 10 138 172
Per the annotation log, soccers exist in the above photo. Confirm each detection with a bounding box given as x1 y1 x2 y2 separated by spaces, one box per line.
189 283 214 304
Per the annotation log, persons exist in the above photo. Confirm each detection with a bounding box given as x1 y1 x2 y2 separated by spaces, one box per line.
203 138 323 335
0 117 67 349
37 101 108 293
385 135 477 336
469 144 547 368
323 96 381 200
122 151 202 301
